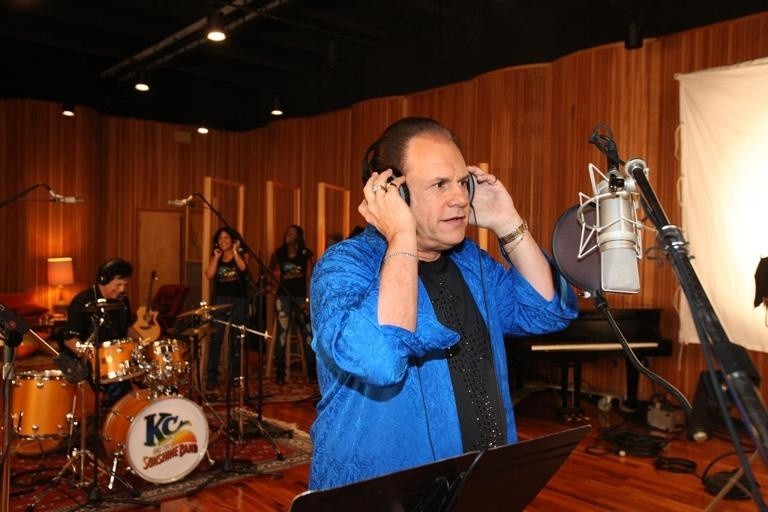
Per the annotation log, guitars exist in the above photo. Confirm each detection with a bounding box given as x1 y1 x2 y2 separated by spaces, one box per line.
133 270 160 342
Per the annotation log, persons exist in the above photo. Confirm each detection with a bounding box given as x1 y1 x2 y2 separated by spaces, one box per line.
203 225 250 397
52 256 134 457
258 223 319 387
303 116 583 494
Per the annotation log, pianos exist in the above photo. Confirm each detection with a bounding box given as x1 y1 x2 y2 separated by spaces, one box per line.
520 308 672 417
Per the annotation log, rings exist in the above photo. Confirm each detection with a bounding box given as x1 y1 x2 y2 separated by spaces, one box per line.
371 185 386 193
489 176 498 186
385 182 399 192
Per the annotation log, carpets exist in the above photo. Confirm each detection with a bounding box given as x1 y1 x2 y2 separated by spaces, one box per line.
0 407 313 512
179 350 318 406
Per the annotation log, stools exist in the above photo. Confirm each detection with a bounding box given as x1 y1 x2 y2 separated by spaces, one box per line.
265 312 308 382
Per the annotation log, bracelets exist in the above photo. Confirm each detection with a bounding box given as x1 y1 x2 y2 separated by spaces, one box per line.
378 247 421 267
495 218 530 256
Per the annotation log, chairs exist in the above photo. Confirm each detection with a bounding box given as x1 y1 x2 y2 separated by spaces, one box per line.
0 291 49 326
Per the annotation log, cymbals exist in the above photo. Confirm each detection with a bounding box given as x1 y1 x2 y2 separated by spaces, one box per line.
180 301 231 314
77 300 120 315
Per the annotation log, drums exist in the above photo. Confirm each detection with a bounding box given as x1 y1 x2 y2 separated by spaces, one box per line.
90 337 151 383
11 369 77 455
141 340 190 380
102 387 209 484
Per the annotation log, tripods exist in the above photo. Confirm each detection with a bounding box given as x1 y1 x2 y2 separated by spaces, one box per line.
53 379 111 485
185 288 284 500
210 330 283 460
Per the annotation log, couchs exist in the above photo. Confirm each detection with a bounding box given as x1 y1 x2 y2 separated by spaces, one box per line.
151 283 190 316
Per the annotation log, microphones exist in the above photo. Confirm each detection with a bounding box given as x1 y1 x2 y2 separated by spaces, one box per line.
49 189 85 203
167 194 193 207
552 128 641 295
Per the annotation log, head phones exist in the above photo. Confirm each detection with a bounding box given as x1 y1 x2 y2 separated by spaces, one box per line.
361 144 475 205
96 259 119 285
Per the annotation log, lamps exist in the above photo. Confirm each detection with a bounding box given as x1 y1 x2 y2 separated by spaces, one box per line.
61 101 75 117
47 256 75 313
206 10 228 44
270 96 286 117
